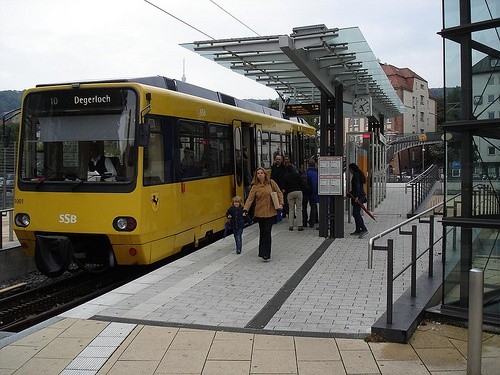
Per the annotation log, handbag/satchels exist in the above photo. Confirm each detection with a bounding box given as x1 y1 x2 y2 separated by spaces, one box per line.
271 192 283 209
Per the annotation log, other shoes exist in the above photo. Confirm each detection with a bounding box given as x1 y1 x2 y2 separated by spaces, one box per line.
298 227 304 231
350 229 369 238
258 256 272 261
236 247 242 254
289 226 293 230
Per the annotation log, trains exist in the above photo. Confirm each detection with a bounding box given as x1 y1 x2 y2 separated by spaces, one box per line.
12 75 316 278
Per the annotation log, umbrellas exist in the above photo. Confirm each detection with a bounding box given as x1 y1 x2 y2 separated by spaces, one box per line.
223 219 230 240
349 193 377 223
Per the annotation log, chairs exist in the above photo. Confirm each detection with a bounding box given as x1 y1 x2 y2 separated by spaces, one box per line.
104 158 120 177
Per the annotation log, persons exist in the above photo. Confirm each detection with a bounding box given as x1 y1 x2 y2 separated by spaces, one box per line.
226 196 248 254
242 167 284 261
87 144 117 182
346 163 369 238
271 153 318 232
180 147 194 174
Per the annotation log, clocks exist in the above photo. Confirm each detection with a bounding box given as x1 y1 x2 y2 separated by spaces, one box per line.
352 96 372 116
